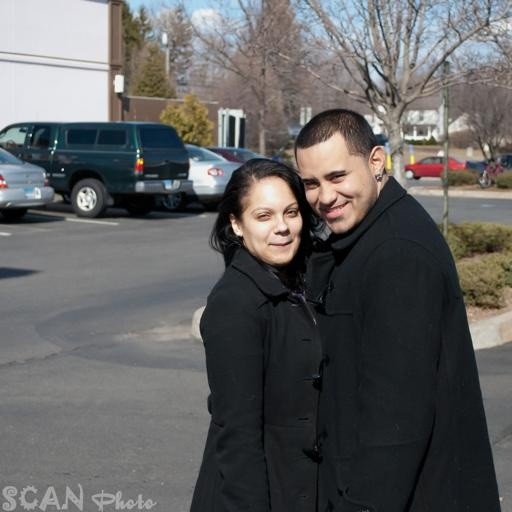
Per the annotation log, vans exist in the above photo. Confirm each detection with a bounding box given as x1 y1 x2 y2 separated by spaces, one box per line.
1 121 195 218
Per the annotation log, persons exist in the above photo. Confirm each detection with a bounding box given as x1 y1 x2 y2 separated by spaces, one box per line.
294 107 501 512
188 156 322 511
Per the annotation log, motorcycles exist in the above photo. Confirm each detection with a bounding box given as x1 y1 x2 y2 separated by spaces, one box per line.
478 165 505 188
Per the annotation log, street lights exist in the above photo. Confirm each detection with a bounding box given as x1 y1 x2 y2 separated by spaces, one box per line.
159 31 176 78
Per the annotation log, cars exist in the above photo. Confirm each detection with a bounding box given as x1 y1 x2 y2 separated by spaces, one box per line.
288 121 303 140
207 147 276 164
467 153 512 176
374 133 388 147
404 157 468 180
0 147 54 222
161 143 244 213
